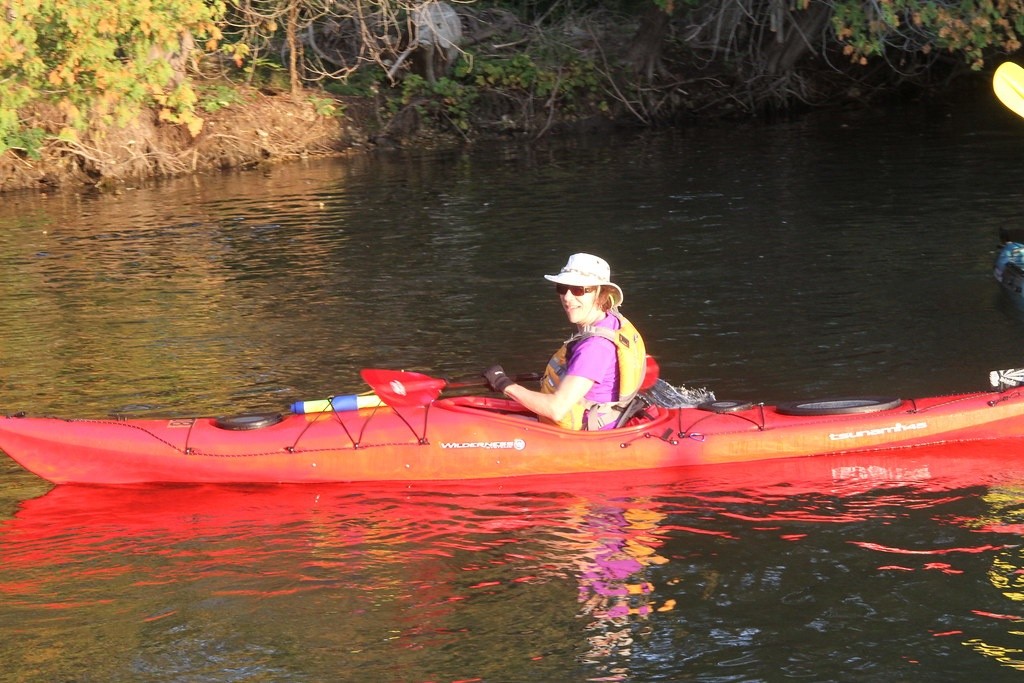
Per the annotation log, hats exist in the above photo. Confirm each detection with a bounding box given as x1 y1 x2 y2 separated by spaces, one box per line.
544 253 624 310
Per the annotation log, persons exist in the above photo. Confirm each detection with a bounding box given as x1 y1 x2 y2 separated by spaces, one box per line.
482 253 646 431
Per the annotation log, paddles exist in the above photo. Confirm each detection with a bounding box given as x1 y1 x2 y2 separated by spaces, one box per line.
357 352 662 411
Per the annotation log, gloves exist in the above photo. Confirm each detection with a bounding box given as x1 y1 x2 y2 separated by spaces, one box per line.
479 364 516 393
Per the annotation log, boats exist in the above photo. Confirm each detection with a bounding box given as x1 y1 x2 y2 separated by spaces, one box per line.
0 376 1024 488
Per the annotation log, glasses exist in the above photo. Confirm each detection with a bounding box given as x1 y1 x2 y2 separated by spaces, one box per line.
554 283 597 296
